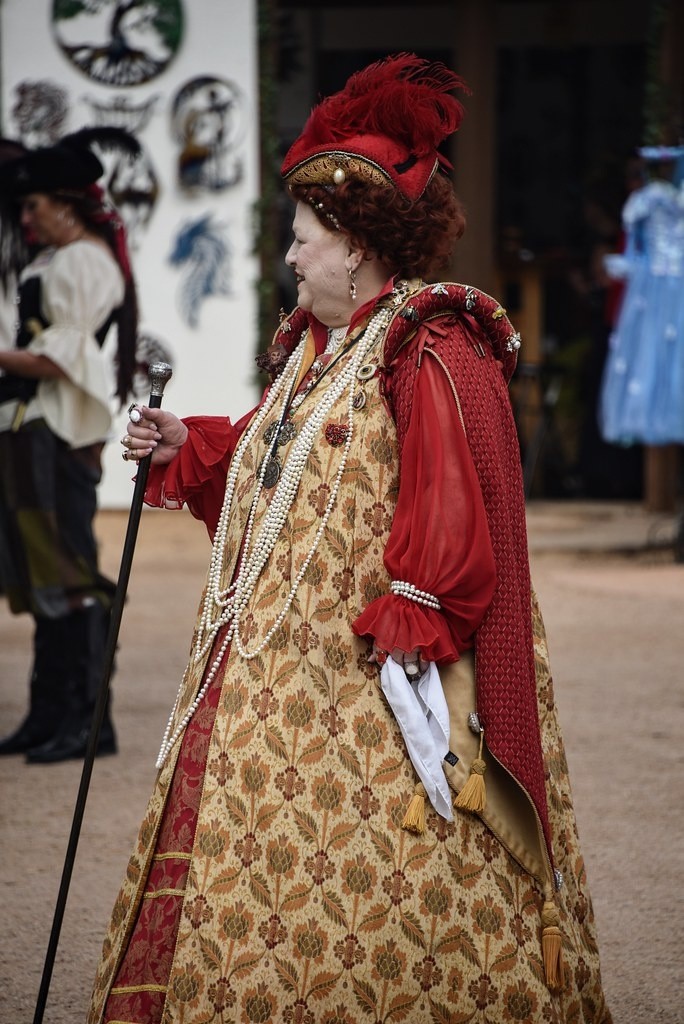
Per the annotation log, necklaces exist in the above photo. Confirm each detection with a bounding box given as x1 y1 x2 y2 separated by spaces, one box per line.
153 275 414 771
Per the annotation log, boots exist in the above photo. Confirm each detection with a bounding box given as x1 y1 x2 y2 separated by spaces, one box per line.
0 575 128 764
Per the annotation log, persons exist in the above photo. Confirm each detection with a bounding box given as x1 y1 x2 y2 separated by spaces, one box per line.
81 55 612 1023
598 106 684 519
0 127 144 768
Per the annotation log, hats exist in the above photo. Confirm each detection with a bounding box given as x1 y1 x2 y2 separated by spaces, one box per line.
278 51 473 203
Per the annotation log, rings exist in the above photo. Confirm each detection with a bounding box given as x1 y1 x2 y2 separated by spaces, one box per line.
119 434 132 448
121 450 128 460
375 648 388 663
402 645 420 653
405 662 420 677
126 402 143 424
131 448 138 459
421 656 428 663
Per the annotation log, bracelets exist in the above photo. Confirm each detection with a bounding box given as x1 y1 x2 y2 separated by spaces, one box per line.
387 580 442 611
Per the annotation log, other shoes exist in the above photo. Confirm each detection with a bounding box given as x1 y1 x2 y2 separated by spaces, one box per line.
645 513 680 549
